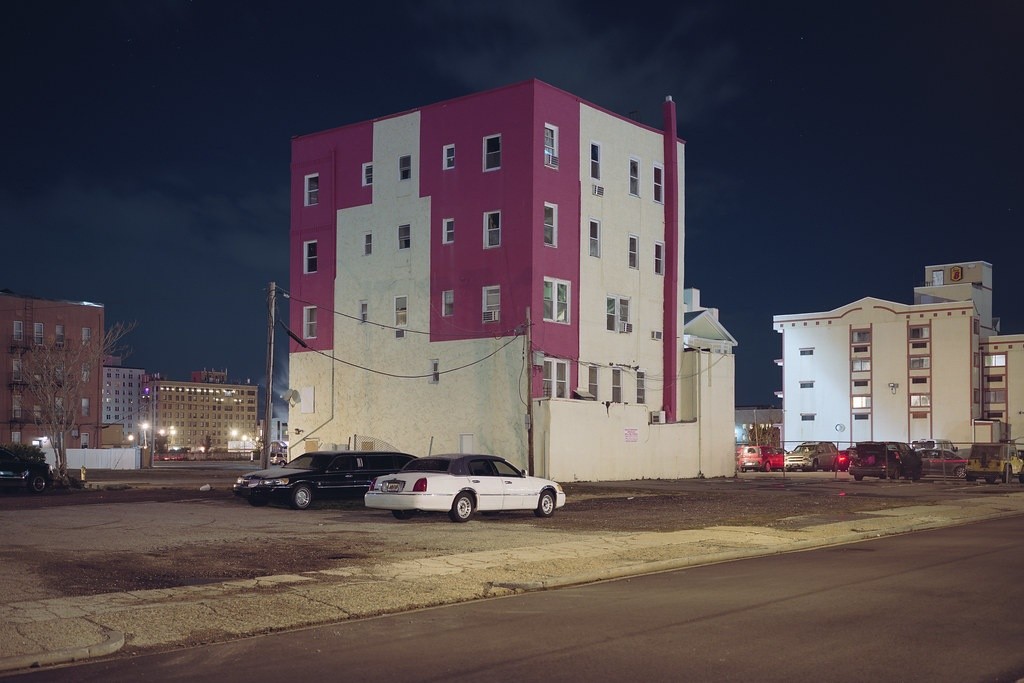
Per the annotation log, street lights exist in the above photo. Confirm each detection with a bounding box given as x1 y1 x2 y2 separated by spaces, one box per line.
242 434 248 456
143 423 149 446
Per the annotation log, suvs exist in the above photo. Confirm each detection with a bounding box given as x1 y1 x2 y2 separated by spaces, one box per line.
784 441 841 472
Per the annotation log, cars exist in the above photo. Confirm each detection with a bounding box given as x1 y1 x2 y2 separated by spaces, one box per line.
736 446 789 472
364 452 566 523
964 442 1024 484
914 449 968 477
0 446 52 494
233 450 419 511
837 450 851 472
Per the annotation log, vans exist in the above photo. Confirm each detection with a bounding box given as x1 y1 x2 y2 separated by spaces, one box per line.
848 440 914 482
912 439 958 453
